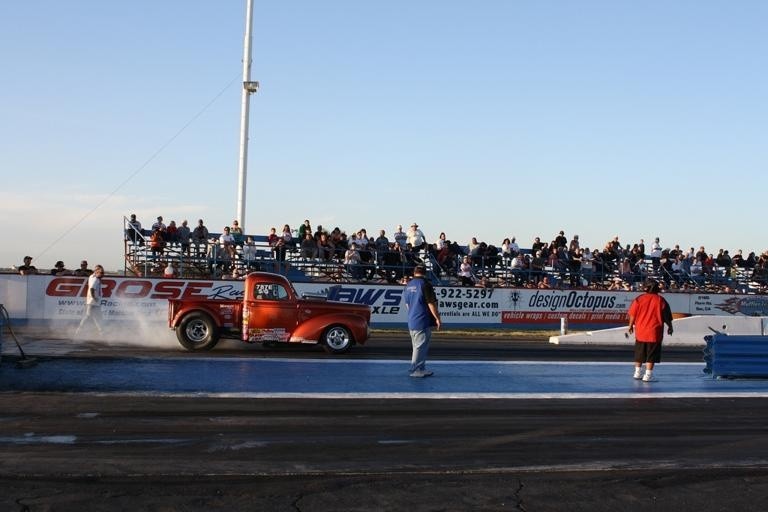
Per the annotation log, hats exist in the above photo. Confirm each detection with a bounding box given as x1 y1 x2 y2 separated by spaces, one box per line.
410 223 419 228
641 278 661 294
414 266 428 274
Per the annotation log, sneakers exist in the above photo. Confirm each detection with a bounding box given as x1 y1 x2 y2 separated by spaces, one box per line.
642 373 660 382
408 369 434 378
632 367 644 380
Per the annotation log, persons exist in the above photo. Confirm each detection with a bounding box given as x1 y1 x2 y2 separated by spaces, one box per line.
625 281 674 383
71 266 106 339
403 267 442 378
18 256 38 276
74 261 94 276
51 261 72 276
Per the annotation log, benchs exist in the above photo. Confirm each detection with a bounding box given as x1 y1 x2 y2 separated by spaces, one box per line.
492 248 768 295
122 229 492 287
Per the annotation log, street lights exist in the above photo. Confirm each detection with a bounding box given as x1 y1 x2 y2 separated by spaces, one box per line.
239 0 256 234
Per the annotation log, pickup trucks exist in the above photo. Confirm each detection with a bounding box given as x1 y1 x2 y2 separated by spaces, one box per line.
166 271 374 352
